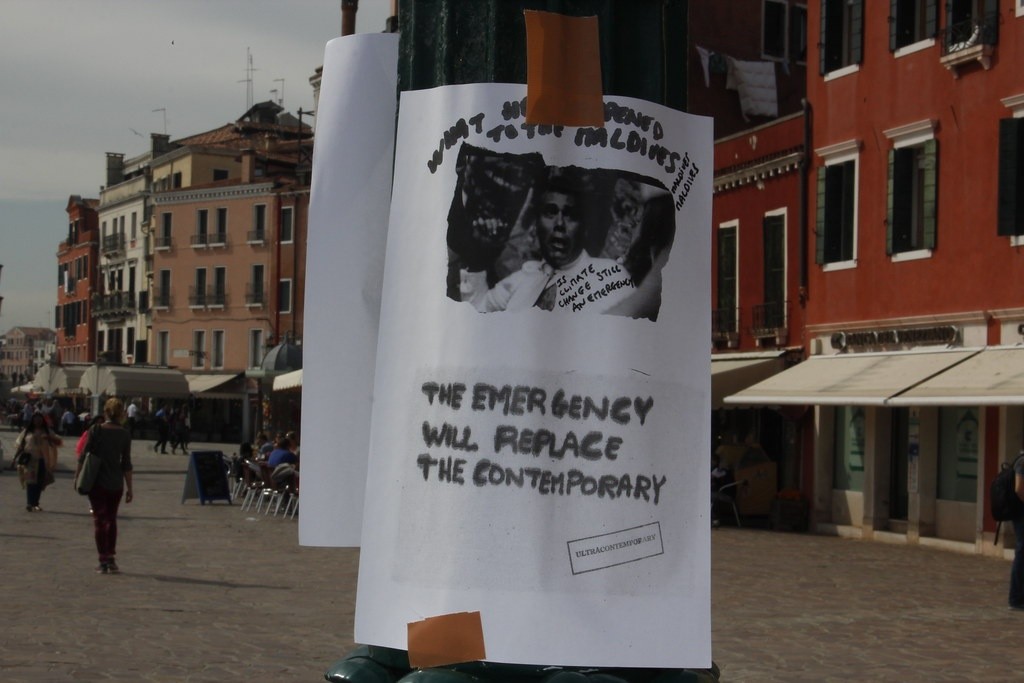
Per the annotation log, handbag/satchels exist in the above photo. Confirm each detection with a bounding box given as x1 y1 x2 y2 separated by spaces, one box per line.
74 423 102 496
17 428 32 466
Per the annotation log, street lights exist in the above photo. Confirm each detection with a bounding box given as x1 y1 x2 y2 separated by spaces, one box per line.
265 107 311 343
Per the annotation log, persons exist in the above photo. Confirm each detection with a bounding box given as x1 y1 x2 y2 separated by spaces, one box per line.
73 397 133 575
1008 449 1024 608
1 386 191 454
461 172 670 318
9 411 62 511
236 426 302 502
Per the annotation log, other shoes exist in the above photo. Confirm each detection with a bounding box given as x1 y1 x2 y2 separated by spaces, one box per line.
25 506 42 512
107 561 120 574
94 562 107 575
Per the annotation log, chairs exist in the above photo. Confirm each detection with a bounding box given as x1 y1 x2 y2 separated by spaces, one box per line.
220 452 300 520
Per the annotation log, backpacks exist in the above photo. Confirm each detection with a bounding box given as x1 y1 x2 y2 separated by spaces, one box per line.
989 453 1024 521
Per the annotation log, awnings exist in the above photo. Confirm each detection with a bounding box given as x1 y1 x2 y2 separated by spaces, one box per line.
711 350 786 411
272 368 302 401
183 373 247 400
724 346 1023 405
